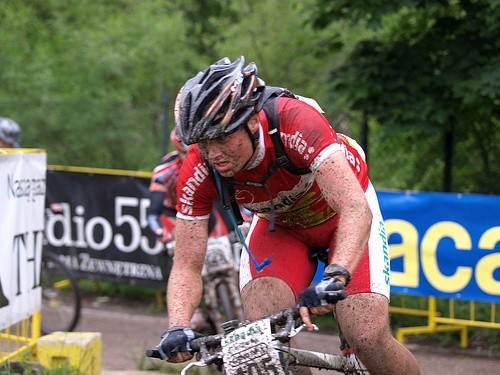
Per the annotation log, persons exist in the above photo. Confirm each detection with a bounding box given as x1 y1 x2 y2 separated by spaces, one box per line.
0 117 22 148
157 54 421 375
149 127 254 332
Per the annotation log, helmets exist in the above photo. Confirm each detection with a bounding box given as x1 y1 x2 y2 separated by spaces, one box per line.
168 128 190 151
0 117 20 142
174 55 260 145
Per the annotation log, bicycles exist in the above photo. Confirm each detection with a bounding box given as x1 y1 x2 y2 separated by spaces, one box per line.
145 231 369 375
42 251 82 335
